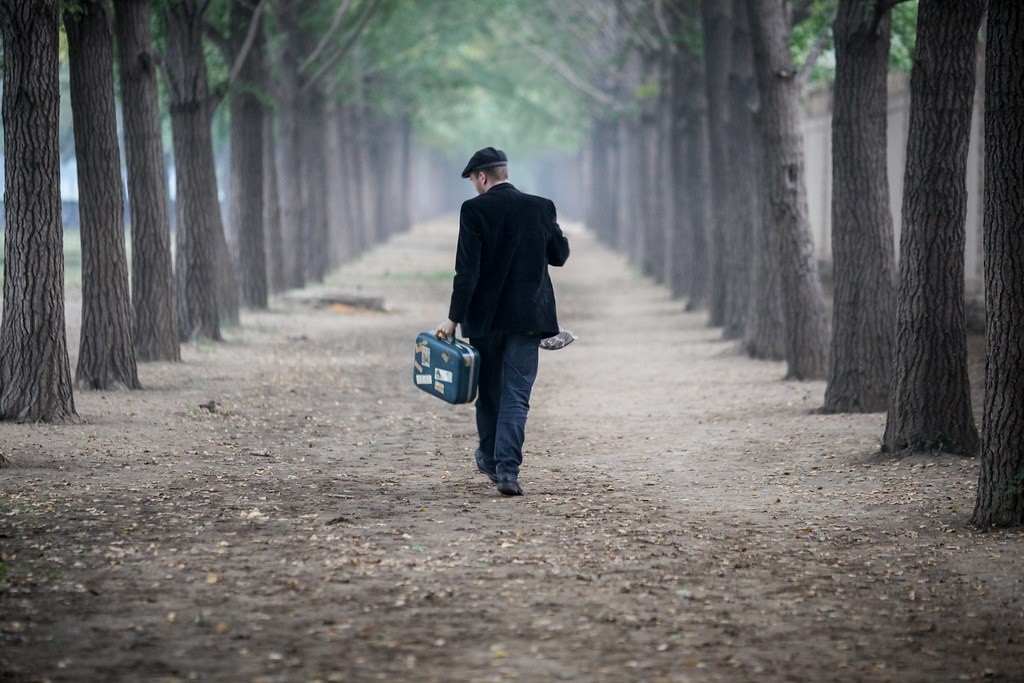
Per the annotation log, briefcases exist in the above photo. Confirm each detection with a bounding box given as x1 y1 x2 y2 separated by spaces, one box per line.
412 331 481 405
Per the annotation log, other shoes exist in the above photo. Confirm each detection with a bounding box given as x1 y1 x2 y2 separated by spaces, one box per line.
475 448 499 483
499 473 525 495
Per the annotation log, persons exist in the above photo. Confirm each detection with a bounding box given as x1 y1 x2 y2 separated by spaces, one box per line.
434 147 570 495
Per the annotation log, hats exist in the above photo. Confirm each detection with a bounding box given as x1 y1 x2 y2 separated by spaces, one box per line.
461 147 508 178
